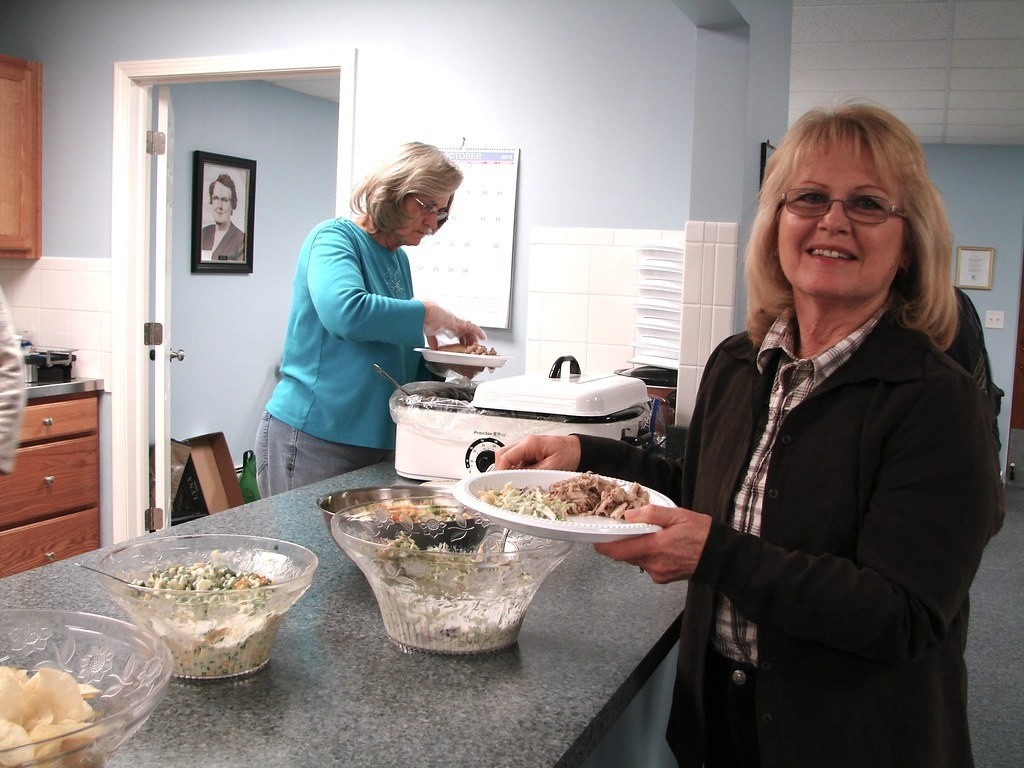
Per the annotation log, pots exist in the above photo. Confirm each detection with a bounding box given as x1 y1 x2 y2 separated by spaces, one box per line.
614 366 678 436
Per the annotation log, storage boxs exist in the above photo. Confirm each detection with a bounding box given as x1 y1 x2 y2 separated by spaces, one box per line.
171 431 246 528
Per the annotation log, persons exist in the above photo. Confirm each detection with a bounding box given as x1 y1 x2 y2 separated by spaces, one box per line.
946 286 1004 452
201 174 245 262
495 106 1005 768
255 142 494 499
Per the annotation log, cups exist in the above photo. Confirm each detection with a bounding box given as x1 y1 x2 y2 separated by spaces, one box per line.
25 364 38 382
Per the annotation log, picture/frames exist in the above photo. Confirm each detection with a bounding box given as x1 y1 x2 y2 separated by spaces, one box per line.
955 246 995 290
190 150 257 274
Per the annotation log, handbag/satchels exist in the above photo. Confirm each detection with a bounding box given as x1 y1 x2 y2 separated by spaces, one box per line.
239 450 261 504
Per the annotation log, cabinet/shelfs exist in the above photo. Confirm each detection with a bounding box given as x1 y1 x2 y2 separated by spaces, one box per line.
0 54 44 260
0 396 99 578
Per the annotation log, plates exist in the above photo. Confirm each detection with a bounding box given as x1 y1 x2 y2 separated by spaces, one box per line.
414 347 513 367
452 469 678 543
421 481 459 486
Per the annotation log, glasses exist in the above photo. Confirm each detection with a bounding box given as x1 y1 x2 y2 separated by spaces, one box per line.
778 188 909 224
211 195 231 204
411 193 448 221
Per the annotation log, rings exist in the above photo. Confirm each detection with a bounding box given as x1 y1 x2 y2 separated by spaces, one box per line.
639 566 644 574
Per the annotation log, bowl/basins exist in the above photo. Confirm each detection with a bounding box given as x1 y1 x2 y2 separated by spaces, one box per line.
0 610 174 768
331 495 574 655
316 485 489 565
97 534 318 680
